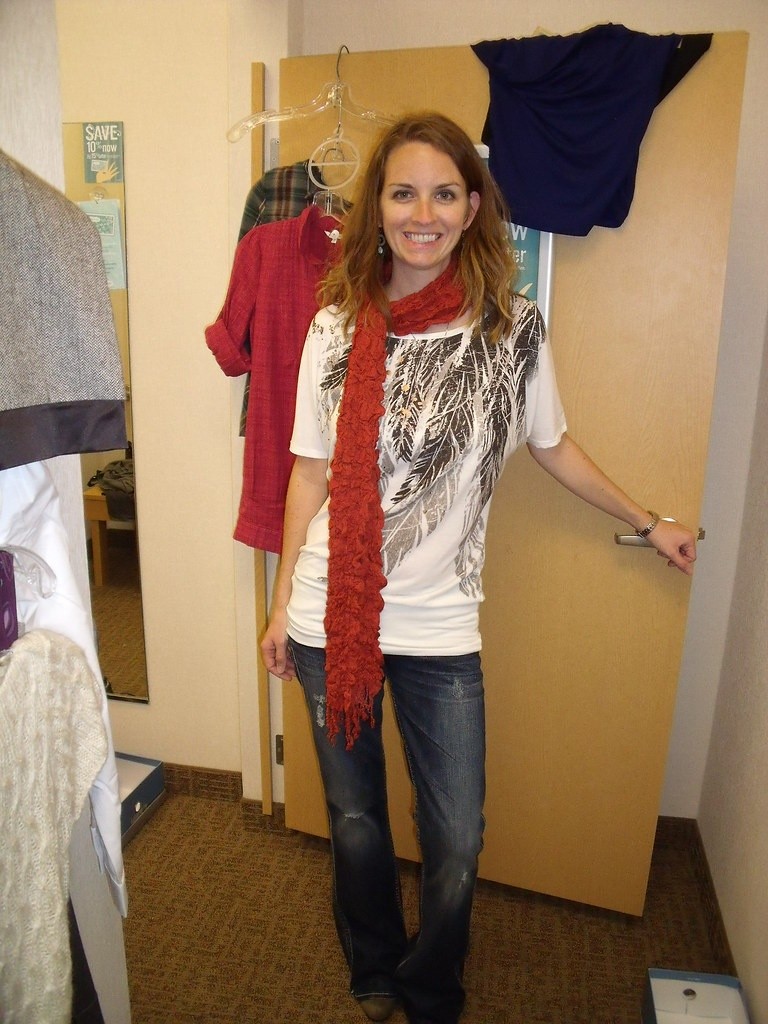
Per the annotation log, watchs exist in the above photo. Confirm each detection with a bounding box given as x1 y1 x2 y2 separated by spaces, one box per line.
635 510 660 538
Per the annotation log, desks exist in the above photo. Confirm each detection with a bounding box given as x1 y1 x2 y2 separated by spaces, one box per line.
85 482 136 586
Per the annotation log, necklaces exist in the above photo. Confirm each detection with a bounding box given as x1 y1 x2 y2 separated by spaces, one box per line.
411 322 450 375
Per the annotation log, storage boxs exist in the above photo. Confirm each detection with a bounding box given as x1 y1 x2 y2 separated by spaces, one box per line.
640 967 750 1024
115 753 168 848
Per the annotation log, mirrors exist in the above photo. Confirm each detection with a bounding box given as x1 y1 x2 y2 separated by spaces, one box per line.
61 121 148 704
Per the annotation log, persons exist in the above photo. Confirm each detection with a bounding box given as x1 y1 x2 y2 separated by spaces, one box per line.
260 112 698 1024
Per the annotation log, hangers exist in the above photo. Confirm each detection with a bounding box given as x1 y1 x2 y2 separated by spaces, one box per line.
226 44 400 227
0 544 58 650
